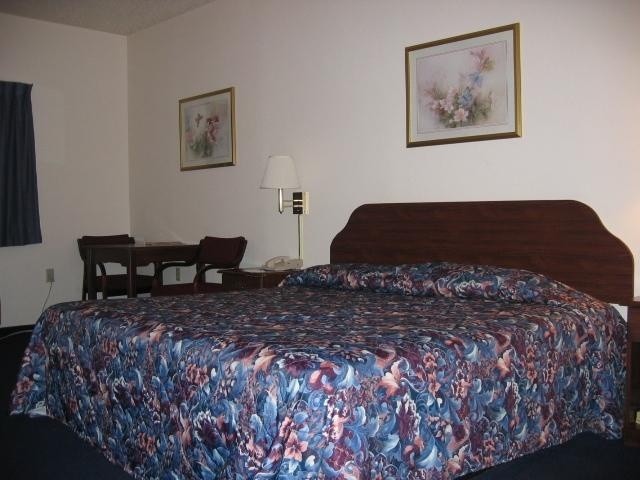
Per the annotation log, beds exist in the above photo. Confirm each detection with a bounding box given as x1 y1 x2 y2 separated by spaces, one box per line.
10 200 634 480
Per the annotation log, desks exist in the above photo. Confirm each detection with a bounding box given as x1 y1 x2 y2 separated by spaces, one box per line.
78 242 200 301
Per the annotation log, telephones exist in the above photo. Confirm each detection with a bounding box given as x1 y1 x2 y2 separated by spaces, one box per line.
260 256 304 271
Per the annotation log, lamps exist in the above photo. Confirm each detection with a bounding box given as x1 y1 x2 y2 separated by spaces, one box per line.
260 155 310 215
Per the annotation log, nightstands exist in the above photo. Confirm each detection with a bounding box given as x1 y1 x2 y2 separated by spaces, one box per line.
619 295 640 446
216 267 303 293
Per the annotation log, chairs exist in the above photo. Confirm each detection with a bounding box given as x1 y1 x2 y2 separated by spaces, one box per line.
151 236 248 297
77 233 153 301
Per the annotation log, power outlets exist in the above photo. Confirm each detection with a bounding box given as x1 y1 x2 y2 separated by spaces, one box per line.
176 268 180 281
46 268 55 282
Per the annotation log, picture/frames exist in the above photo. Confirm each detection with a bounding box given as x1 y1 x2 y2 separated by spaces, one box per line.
178 87 236 171
405 22 523 148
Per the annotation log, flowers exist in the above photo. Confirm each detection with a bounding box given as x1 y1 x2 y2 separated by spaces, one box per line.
420 47 498 128
185 112 223 158
